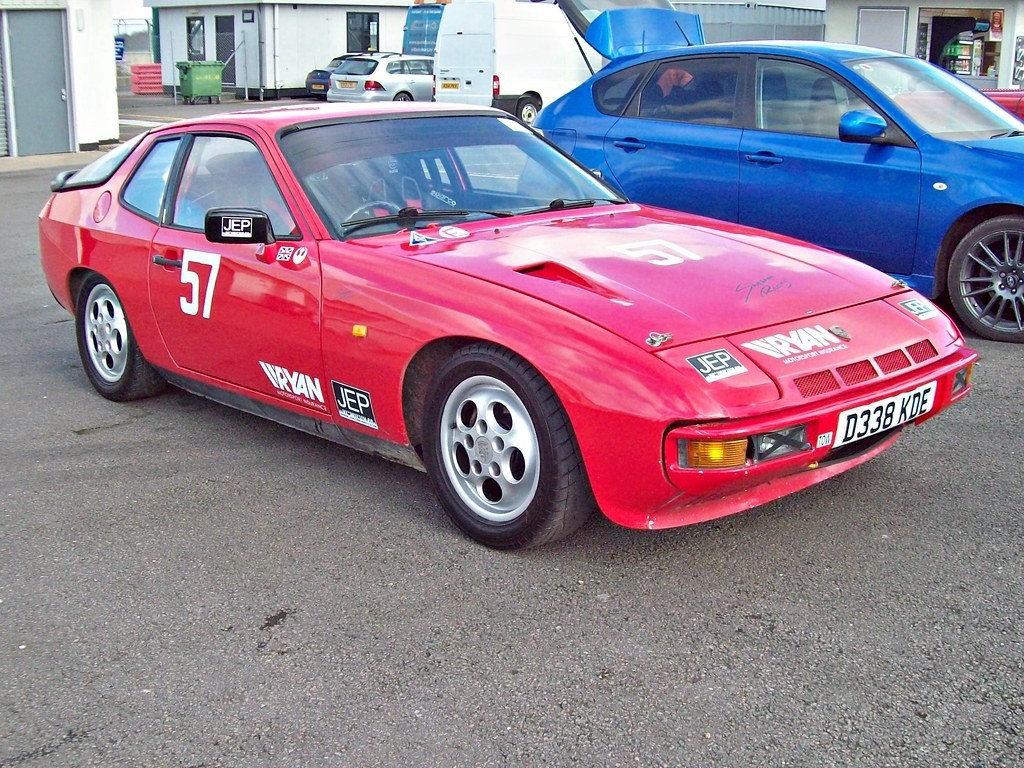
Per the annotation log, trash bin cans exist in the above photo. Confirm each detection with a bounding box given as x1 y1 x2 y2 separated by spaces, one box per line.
175 60 226 105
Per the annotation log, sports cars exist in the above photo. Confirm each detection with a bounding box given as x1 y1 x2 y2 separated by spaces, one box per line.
34 101 980 554
510 6 1024 342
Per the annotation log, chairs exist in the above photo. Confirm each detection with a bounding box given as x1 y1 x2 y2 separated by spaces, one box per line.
763 67 803 133
807 78 840 136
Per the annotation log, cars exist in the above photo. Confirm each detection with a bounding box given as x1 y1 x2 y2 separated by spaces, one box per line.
326 53 433 101
304 52 400 100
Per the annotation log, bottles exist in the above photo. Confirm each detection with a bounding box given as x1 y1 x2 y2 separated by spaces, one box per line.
917 31 927 59
945 59 970 71
945 45 970 56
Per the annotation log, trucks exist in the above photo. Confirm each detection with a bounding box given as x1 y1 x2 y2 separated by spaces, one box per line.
433 0 611 126
400 3 444 74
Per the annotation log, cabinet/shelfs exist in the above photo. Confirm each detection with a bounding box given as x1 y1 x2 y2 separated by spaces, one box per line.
943 40 982 76
